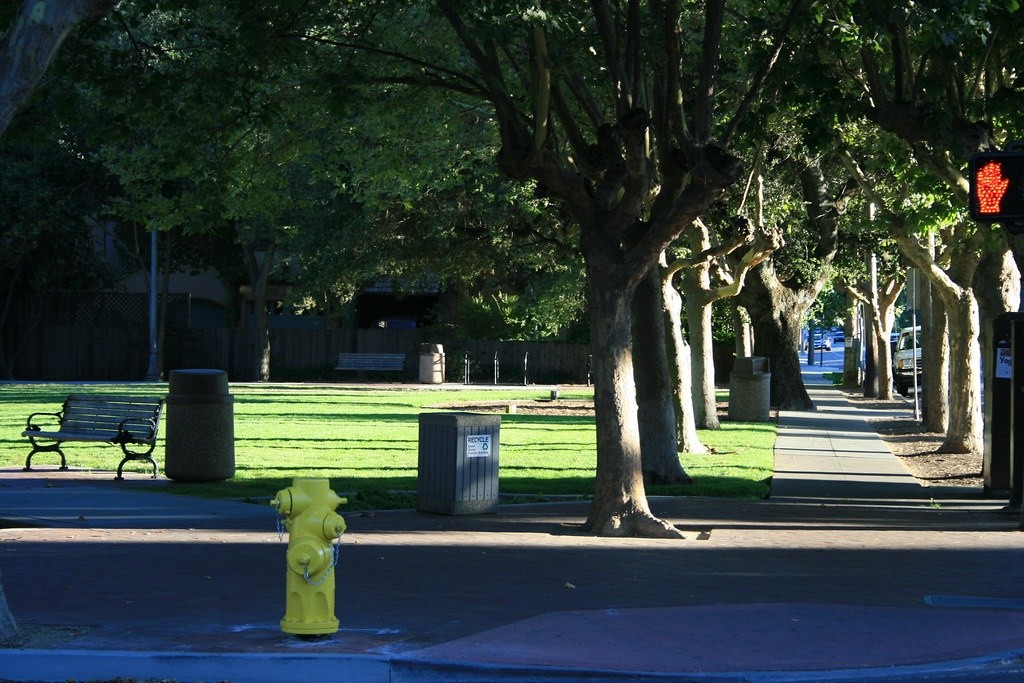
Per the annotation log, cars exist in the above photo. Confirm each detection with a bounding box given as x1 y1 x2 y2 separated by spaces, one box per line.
805 334 831 351
834 331 845 344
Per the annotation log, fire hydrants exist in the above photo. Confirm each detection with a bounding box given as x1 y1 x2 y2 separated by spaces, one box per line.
269 477 349 635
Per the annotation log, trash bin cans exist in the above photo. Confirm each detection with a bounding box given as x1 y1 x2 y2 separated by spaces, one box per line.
416 411 503 515
164 369 235 484
727 356 772 423
418 342 446 384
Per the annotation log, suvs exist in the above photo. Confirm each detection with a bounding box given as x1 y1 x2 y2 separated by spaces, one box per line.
892 326 922 396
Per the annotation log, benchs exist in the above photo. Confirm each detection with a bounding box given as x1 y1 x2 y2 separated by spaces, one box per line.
22 395 165 481
334 353 406 382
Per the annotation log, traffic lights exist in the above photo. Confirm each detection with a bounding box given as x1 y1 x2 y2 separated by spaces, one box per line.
967 151 1024 224
